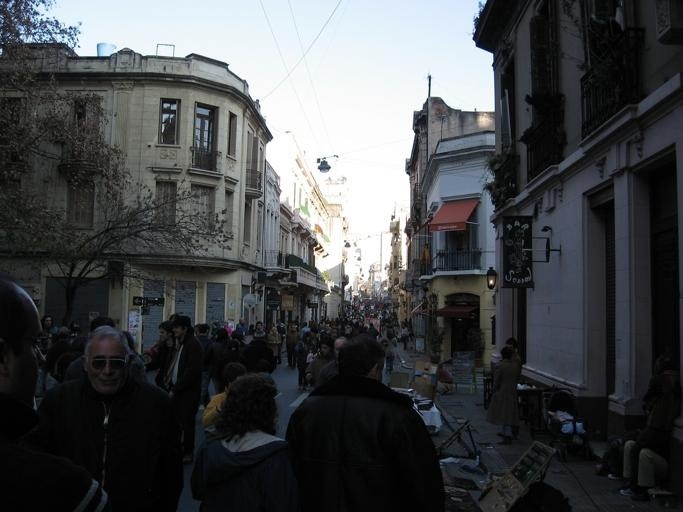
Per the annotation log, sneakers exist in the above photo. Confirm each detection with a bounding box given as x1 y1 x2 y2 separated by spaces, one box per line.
297 385 315 392
607 473 651 502
184 452 195 464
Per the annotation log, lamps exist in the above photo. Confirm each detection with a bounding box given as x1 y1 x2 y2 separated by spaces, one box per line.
484 265 498 291
315 155 331 173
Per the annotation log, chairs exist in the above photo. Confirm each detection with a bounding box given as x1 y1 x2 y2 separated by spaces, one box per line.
390 368 587 511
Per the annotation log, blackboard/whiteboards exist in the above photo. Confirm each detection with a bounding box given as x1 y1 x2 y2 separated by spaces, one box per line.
508 441 557 492
452 351 476 384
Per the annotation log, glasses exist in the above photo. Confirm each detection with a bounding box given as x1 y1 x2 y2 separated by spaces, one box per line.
90 351 128 370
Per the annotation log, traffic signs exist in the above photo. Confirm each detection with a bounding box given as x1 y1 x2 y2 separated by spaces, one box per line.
132 296 163 306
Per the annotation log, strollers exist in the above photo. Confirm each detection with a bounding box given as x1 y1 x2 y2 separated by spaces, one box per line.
538 383 591 454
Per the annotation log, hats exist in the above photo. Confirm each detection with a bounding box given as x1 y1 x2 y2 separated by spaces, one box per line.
172 315 194 334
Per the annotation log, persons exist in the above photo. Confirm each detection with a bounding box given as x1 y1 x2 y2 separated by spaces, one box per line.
488 346 519 446
608 355 682 502
505 338 522 364
